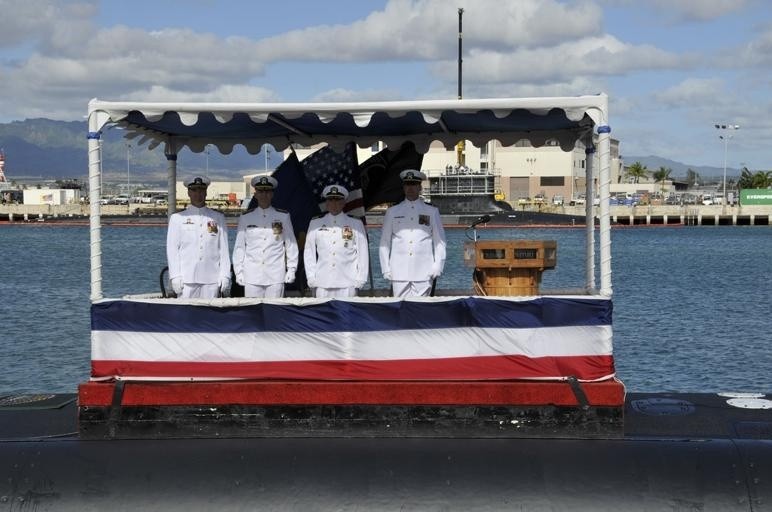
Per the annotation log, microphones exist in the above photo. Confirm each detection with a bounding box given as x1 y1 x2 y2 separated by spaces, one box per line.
468 214 491 229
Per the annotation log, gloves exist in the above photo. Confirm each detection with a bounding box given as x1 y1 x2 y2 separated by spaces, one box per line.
218 277 230 293
285 271 295 284
171 277 184 295
236 272 245 286
354 280 367 289
307 277 316 289
383 272 391 282
429 260 443 280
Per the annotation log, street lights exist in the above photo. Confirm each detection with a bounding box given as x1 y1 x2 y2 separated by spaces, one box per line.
714 124 741 214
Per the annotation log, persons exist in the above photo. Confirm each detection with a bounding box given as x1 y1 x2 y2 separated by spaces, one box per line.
167 173 231 299
380 169 447 295
231 173 299 296
302 183 370 297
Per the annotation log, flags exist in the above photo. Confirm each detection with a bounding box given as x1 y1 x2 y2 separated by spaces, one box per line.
268 136 368 229
248 149 305 208
350 146 424 203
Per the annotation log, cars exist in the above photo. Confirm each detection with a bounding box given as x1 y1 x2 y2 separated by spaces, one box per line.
516 191 741 205
98 192 172 206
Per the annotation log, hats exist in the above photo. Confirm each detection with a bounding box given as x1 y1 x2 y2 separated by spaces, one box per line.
400 169 426 182
322 185 349 201
251 175 278 191
184 174 211 189
